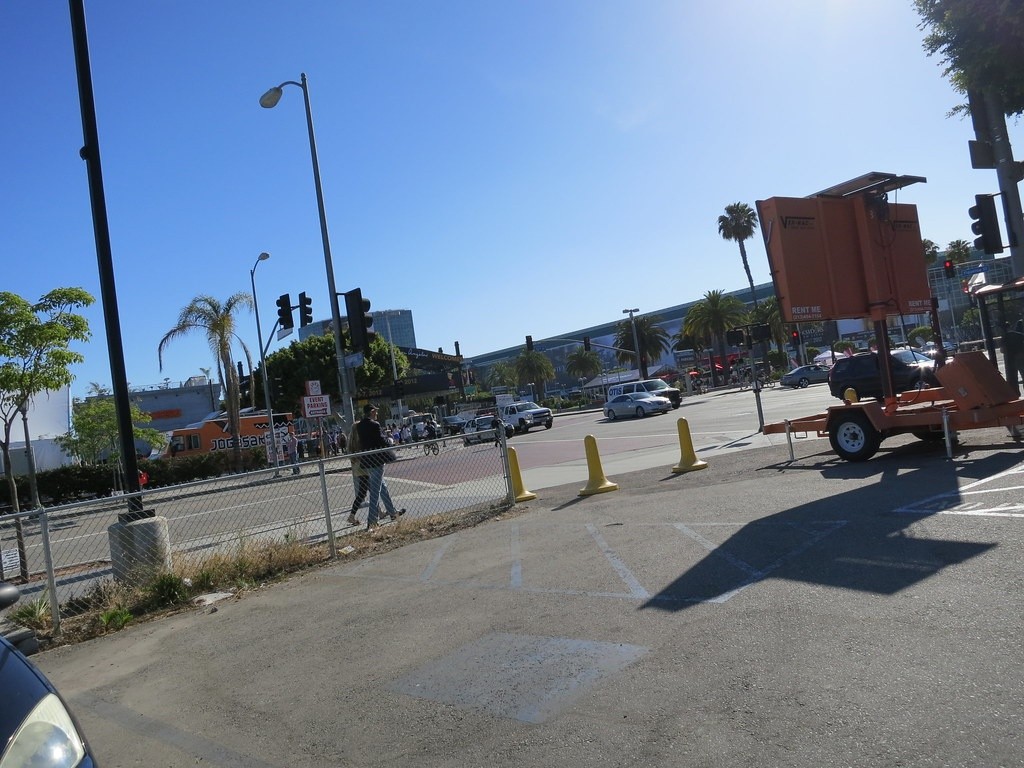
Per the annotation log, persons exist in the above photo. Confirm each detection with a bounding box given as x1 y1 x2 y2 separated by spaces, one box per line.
137 469 143 491
491 414 502 447
347 421 390 526
286 432 300 475
556 402 561 413
695 376 703 395
382 423 419 451
1000 319 1024 396
790 358 799 388
297 431 347 463
424 421 436 448
357 404 406 528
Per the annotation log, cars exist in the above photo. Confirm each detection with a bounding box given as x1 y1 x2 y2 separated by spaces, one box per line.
602 391 673 420
828 348 945 404
779 364 831 389
399 411 516 446
895 340 956 359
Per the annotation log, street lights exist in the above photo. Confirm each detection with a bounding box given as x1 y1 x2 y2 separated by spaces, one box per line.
256 71 369 509
623 308 644 381
250 251 283 478
577 376 587 405
597 373 607 403
526 382 535 402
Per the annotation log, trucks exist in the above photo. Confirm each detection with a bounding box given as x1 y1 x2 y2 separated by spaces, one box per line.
145 411 297 467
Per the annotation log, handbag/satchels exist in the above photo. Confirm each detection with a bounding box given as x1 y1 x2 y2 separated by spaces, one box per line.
371 448 397 466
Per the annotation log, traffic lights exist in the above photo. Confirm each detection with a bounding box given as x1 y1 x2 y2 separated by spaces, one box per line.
526 336 534 350
584 336 591 351
276 293 293 328
344 288 376 350
299 291 312 325
943 259 955 279
967 193 1004 255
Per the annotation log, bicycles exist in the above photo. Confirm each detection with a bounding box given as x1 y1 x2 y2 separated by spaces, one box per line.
422 433 439 456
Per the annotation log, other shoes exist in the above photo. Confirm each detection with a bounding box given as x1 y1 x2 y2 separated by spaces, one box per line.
367 522 382 530
390 509 406 520
379 509 389 520
347 515 360 526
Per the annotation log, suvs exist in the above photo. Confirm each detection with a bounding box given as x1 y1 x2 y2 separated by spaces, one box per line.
499 401 554 434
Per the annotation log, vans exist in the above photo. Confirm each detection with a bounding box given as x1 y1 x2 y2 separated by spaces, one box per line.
608 378 683 409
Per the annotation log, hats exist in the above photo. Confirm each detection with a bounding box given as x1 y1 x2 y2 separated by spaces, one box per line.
364 403 380 413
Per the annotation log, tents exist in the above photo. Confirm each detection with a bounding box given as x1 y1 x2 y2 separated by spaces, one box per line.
578 364 675 407
813 349 845 361
